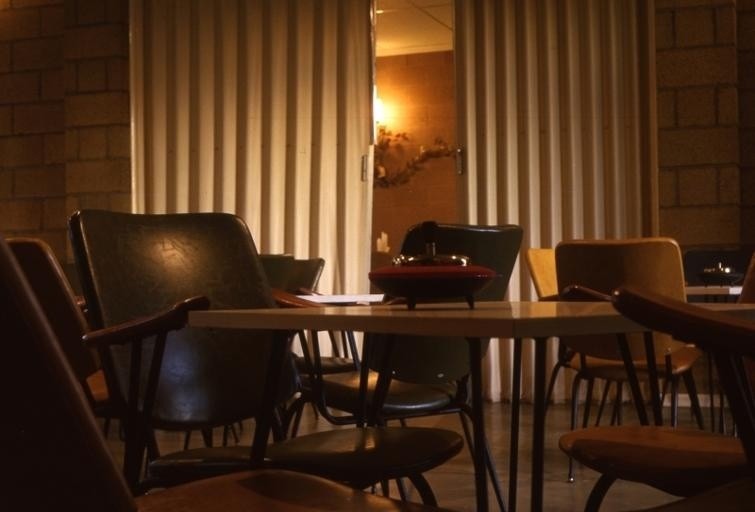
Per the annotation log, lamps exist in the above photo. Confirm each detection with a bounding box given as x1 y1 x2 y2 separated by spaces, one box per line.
373 98 452 191
